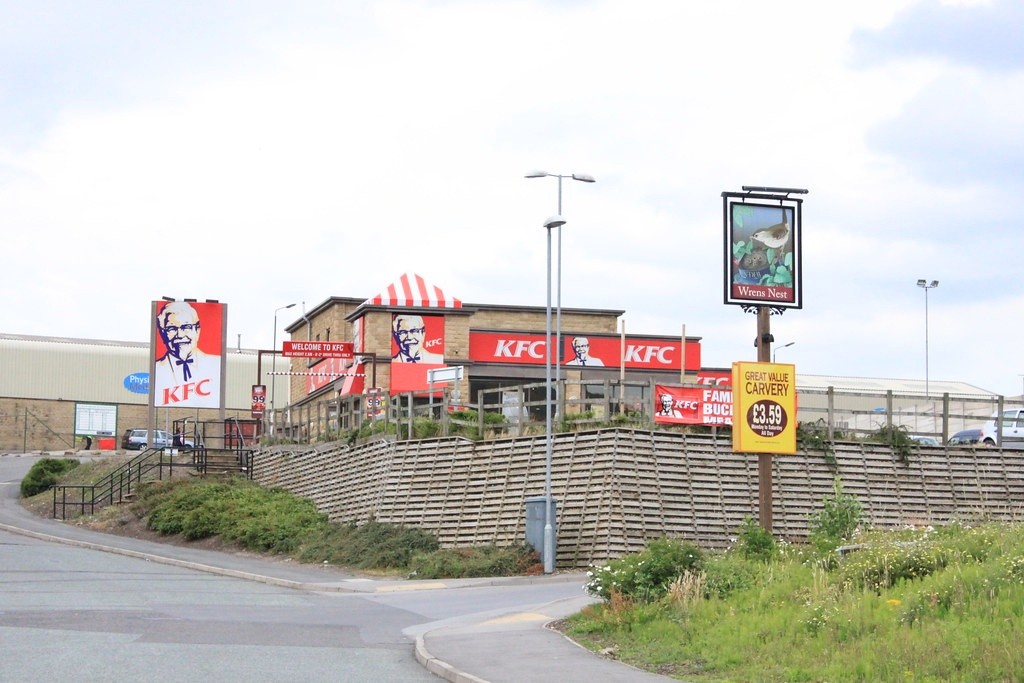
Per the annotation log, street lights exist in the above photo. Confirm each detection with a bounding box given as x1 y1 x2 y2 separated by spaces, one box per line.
917 280 939 395
525 171 596 421
773 342 794 363
1019 374 1024 396
543 216 566 572
272 303 295 409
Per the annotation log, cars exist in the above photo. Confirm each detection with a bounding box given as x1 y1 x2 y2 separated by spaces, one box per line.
978 409 1024 448
121 428 195 450
948 429 980 445
911 437 939 446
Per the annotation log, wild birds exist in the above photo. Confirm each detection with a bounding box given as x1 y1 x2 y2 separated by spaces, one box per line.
751 249 767 267
750 207 790 254
